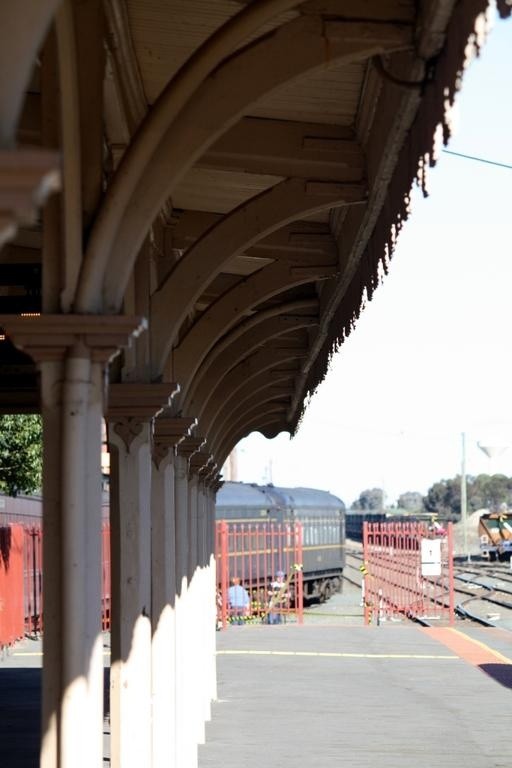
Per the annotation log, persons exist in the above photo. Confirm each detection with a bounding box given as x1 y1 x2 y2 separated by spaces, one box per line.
268 571 290 623
228 577 250 625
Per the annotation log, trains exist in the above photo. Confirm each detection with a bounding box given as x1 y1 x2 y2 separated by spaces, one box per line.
345 509 511 560
0 467 349 664
